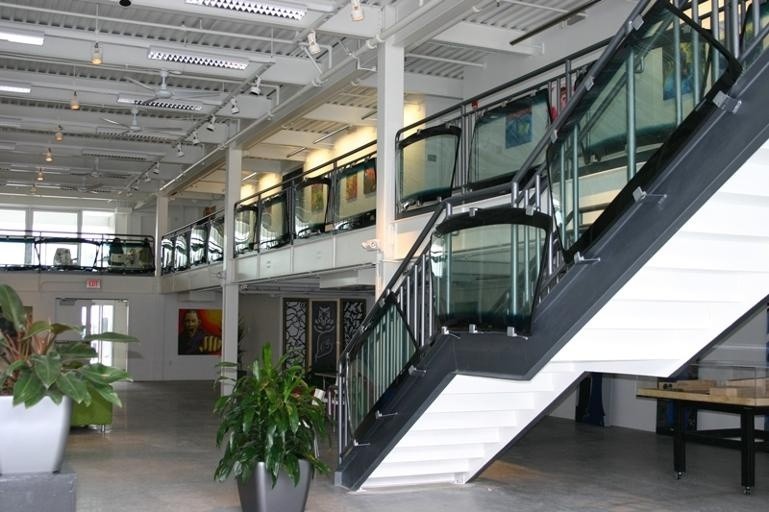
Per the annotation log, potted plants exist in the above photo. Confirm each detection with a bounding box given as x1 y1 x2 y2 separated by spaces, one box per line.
211 347 336 512
0 281 141 474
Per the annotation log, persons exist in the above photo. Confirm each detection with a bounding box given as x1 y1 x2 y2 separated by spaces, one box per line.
180 309 225 354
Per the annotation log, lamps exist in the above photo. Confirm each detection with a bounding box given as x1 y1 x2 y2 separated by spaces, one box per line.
225 73 264 115
301 0 365 54
71 4 102 111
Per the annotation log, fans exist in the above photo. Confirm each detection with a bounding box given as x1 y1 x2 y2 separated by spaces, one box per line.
98 68 222 137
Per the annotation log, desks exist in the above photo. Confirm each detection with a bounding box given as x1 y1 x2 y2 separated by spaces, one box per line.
637 389 769 496
